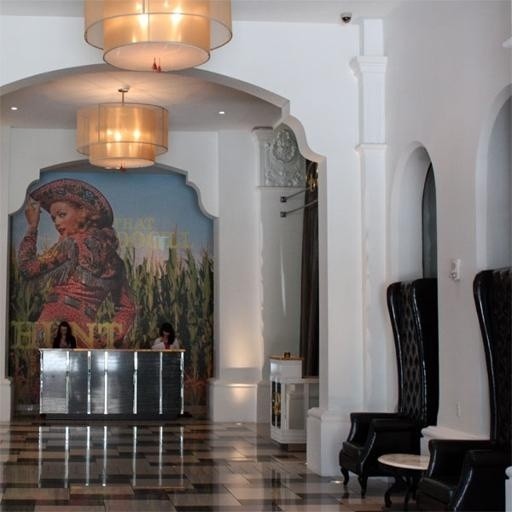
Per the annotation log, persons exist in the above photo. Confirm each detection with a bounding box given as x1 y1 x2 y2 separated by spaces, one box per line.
151 322 180 349
53 321 76 348
15 177 137 427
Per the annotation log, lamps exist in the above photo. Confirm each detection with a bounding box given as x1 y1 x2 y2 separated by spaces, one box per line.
75 0 232 170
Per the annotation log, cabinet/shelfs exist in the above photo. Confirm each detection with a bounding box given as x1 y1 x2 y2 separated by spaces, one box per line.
271 353 319 451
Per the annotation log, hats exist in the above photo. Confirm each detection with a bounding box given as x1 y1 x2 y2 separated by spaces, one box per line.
28 178 114 228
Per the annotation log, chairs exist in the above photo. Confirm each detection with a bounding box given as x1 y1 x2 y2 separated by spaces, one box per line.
339 279 436 500
413 266 511 511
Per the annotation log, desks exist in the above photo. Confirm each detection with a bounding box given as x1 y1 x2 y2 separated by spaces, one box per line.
379 454 436 506
